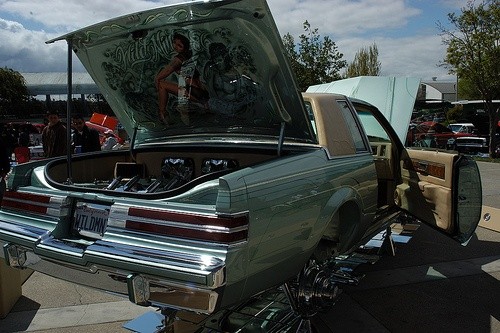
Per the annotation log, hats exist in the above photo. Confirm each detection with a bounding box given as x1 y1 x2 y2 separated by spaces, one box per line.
103 129 113 135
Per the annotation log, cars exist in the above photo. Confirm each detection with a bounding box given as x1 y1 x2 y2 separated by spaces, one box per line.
0 0 488 320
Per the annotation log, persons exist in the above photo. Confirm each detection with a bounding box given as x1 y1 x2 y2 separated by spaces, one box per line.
99 128 128 152
0 117 48 208
42 109 71 158
72 113 101 153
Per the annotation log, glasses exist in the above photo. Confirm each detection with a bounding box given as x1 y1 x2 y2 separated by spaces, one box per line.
73 121 84 125
